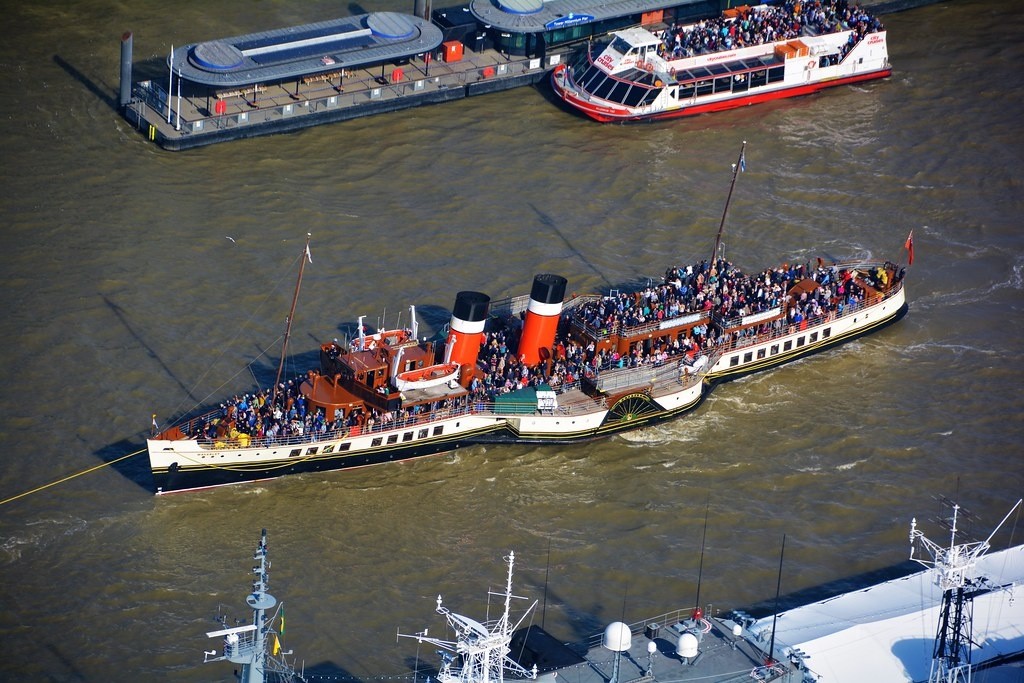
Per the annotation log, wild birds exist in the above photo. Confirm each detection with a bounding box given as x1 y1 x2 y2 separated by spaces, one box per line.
226 236 236 243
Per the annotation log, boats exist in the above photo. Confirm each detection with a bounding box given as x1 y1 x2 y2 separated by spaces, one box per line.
349 328 413 352
145 141 908 495
395 363 460 391
201 499 1024 683
548 5 895 123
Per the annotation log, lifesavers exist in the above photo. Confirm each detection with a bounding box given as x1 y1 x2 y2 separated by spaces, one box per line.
646 62 654 72
670 67 676 76
637 60 644 69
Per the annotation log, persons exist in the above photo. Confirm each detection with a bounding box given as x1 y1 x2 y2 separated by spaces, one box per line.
654 0 885 69
196 257 889 446
899 267 905 281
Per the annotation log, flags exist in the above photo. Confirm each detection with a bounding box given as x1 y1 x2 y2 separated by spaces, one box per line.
905 232 914 264
